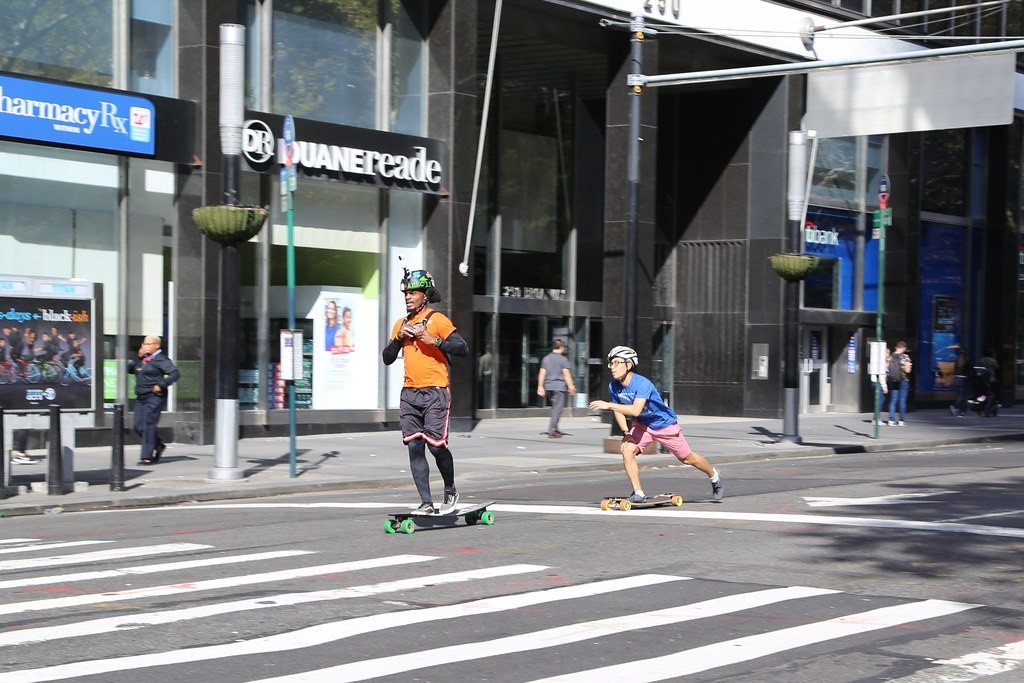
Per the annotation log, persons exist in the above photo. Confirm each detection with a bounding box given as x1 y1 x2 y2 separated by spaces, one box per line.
325 301 355 352
472 344 491 409
537 339 576 438
0 327 86 383
871 340 999 427
381 270 470 516
589 346 725 503
127 335 181 466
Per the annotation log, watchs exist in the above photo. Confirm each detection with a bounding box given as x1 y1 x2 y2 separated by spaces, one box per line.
433 337 442 346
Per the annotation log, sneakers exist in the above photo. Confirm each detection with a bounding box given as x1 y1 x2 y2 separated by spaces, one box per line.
711 467 724 499
438 491 459 515
411 503 435 515
625 492 646 503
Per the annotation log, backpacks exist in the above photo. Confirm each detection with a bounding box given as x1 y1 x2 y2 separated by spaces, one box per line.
976 359 989 386
889 354 903 383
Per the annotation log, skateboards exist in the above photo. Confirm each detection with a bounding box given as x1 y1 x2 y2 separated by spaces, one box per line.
383 500 497 534
600 492 683 511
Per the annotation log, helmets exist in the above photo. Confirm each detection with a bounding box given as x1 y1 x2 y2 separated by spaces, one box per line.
607 346 639 369
400 270 435 292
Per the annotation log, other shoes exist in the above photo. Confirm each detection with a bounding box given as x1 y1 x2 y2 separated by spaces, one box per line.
137 459 152 466
898 421 906 427
153 443 166 462
957 413 967 418
888 420 897 426
548 430 561 438
950 405 957 416
871 419 887 425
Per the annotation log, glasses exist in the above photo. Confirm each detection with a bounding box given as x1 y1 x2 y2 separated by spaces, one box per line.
607 362 631 369
141 343 159 347
